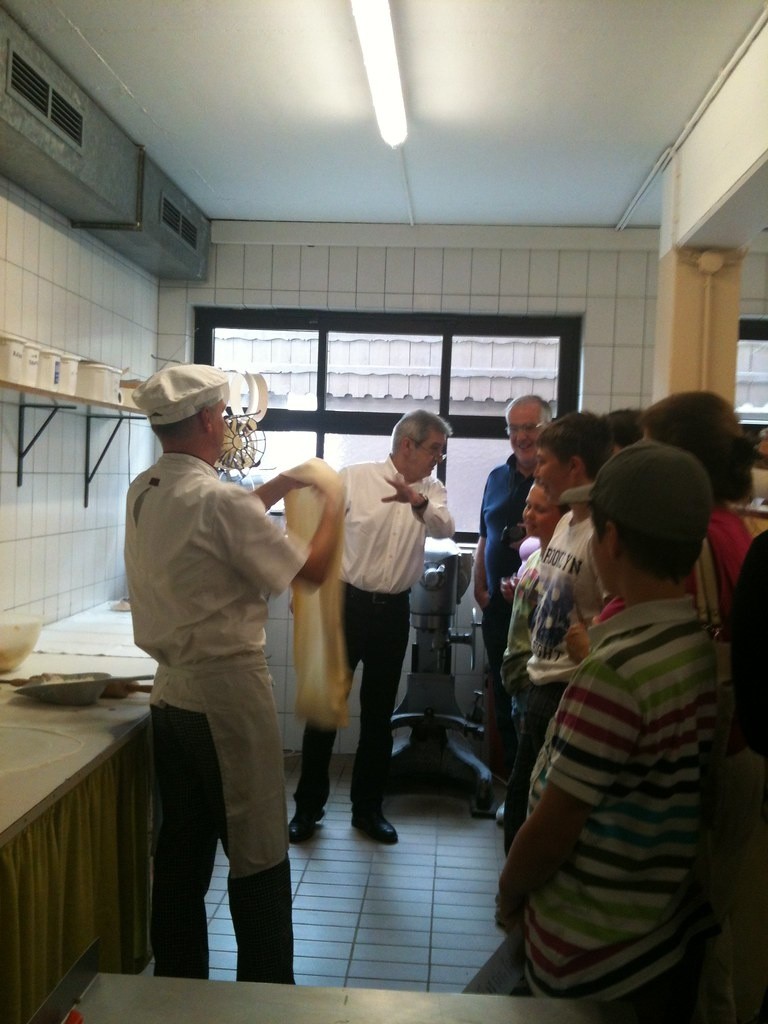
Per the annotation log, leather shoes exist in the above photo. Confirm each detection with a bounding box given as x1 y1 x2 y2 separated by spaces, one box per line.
289 803 325 842
349 809 399 844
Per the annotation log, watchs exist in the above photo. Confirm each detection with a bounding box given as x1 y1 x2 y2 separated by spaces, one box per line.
411 493 429 510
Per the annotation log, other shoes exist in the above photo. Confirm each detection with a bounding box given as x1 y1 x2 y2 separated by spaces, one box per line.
495 800 504 825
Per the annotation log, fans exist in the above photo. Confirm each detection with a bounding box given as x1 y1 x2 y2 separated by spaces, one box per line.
216 414 266 493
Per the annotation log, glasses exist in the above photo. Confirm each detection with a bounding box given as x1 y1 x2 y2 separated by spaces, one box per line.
503 421 544 437
407 435 448 464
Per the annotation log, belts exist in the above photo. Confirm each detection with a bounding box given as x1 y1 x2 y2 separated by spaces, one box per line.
342 583 412 605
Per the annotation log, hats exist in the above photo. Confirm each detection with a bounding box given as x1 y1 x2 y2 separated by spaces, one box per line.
132 364 230 426
559 439 711 540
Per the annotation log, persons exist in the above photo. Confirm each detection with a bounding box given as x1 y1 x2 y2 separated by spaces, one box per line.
124 364 346 985
473 391 768 1024
288 409 454 845
